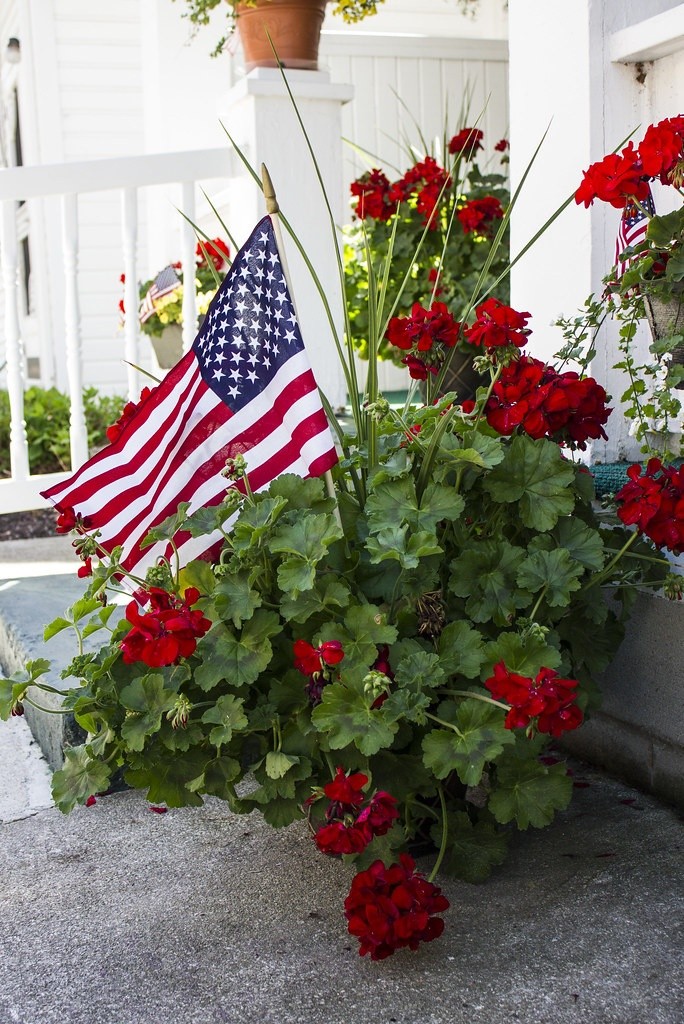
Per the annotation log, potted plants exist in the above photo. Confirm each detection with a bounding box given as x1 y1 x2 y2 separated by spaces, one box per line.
170 0 386 74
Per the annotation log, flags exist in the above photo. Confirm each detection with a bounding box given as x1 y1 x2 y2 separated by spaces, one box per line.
607 162 657 300
139 266 183 322
39 217 339 610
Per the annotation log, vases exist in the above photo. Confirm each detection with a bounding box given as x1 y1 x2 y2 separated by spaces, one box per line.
643 280 684 389
148 319 203 369
419 345 480 406
409 771 478 859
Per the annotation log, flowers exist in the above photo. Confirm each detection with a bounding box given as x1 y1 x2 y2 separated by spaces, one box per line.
574 114 684 295
342 59 511 368
116 236 231 337
0 31 684 960
